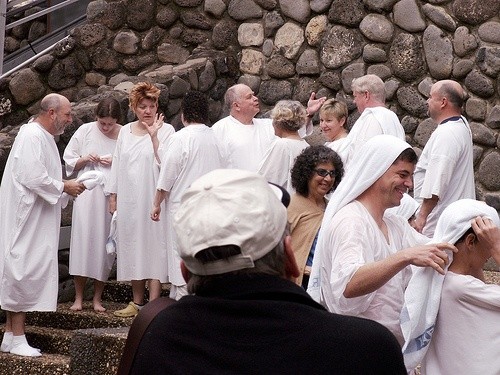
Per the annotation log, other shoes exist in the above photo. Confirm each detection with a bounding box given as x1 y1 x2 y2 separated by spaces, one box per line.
113 302 142 318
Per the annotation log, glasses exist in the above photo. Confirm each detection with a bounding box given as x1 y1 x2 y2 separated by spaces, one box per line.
314 168 338 178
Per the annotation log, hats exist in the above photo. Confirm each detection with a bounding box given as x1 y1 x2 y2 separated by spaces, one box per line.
172 167 291 277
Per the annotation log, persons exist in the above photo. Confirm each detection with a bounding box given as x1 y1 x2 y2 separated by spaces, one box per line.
64 74 476 349
116 169 408 375
0 94 86 358
402 199 500 375
